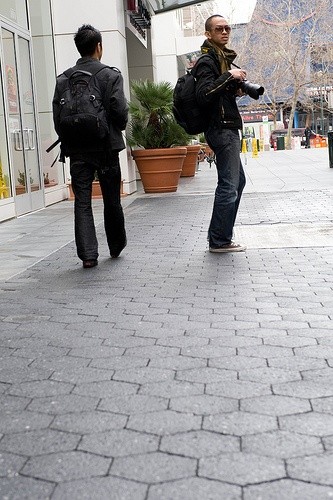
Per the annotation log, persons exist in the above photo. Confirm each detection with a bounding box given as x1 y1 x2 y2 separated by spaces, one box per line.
190 14 247 254
51 24 130 269
303 125 312 149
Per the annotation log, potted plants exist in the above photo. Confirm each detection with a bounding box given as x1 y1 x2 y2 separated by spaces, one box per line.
15 168 40 195
124 77 214 193
44 172 58 188
66 170 127 201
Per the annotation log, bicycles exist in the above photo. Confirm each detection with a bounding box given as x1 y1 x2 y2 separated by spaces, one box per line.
197 141 216 168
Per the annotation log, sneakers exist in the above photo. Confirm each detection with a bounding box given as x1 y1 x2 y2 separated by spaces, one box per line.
208 240 246 252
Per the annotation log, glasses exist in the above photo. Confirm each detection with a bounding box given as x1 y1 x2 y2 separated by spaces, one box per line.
210 27 231 34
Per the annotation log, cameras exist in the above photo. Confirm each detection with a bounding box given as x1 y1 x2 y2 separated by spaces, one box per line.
230 78 265 100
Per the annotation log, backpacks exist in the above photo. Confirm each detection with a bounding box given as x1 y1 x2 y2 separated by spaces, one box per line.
58 64 110 162
172 54 223 135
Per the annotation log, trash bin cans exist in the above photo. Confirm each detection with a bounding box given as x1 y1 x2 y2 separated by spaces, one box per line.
276 136 284 150
327 131 333 168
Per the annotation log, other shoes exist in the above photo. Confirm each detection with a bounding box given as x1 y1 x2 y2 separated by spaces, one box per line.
111 240 127 258
82 259 99 267
